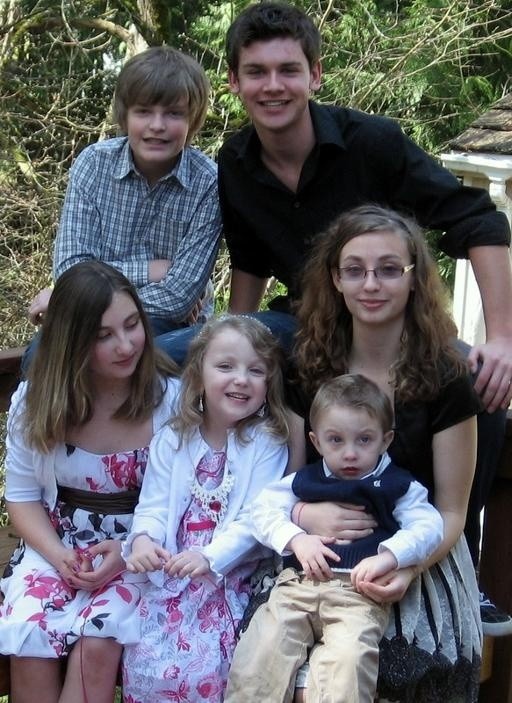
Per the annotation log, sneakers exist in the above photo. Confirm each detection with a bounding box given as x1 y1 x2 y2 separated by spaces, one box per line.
478 591 512 637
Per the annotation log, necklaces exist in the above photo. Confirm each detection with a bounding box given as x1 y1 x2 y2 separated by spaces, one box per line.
192 461 232 524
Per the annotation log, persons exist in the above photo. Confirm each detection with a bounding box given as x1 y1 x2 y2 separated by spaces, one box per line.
1 259 192 703
222 369 448 701
120 313 290 703
147 1 512 640
233 205 485 702
21 47 224 381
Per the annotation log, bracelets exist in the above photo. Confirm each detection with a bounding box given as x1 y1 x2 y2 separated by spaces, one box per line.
294 501 309 526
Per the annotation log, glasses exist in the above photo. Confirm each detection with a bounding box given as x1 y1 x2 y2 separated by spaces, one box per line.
331 262 416 280
